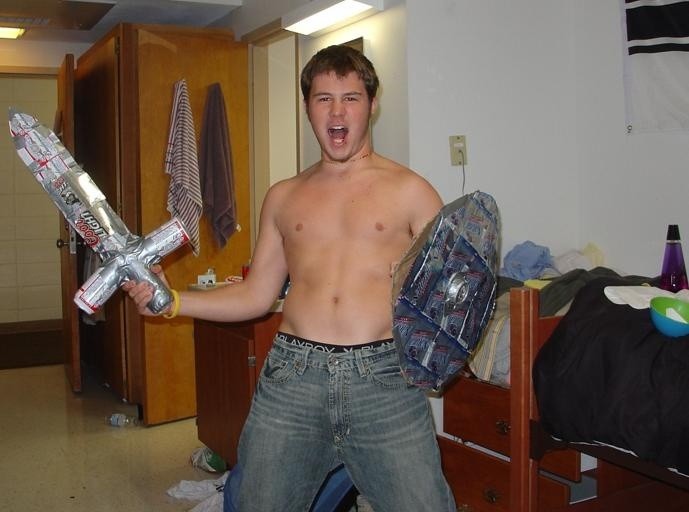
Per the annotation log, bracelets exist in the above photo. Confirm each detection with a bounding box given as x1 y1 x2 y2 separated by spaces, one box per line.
159 287 182 318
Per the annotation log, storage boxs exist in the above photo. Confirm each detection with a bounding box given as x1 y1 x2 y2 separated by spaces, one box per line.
435 373 598 512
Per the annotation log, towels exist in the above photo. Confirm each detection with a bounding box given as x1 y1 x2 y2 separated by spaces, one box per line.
164 77 203 258
201 82 237 250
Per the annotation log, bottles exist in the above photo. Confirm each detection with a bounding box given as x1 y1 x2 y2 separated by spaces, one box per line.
100 412 138 427
658 224 689 293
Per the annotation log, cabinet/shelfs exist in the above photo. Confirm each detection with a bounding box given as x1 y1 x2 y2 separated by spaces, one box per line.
57 20 252 427
187 280 286 470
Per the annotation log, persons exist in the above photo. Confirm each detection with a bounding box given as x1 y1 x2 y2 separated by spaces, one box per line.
121 45 457 511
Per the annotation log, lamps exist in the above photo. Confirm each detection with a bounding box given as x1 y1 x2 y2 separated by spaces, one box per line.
282 0 384 38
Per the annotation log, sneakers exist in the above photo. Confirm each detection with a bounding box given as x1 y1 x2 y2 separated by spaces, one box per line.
190 447 227 473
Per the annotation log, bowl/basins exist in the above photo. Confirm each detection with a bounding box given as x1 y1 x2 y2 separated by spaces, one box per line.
650 296 689 338
